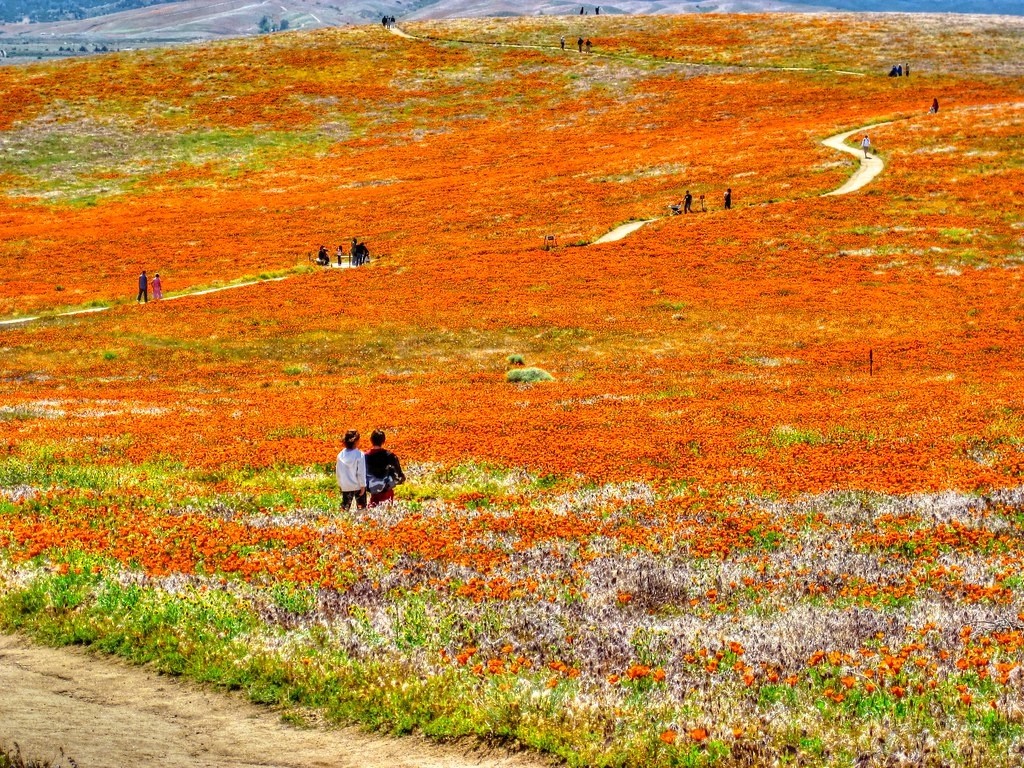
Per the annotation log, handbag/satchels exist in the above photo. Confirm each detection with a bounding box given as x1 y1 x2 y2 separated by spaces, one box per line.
364 475 396 494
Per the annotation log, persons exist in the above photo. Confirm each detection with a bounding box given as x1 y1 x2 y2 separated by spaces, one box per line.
150 274 162 301
337 245 342 266
319 245 329 266
932 98 939 114
351 238 371 267
683 190 694 214
365 430 406 509
862 135 870 159
724 188 732 210
382 15 395 31
561 36 565 50
578 37 584 53
905 62 909 77
888 63 902 77
586 39 592 53
136 271 148 303
335 429 367 512
